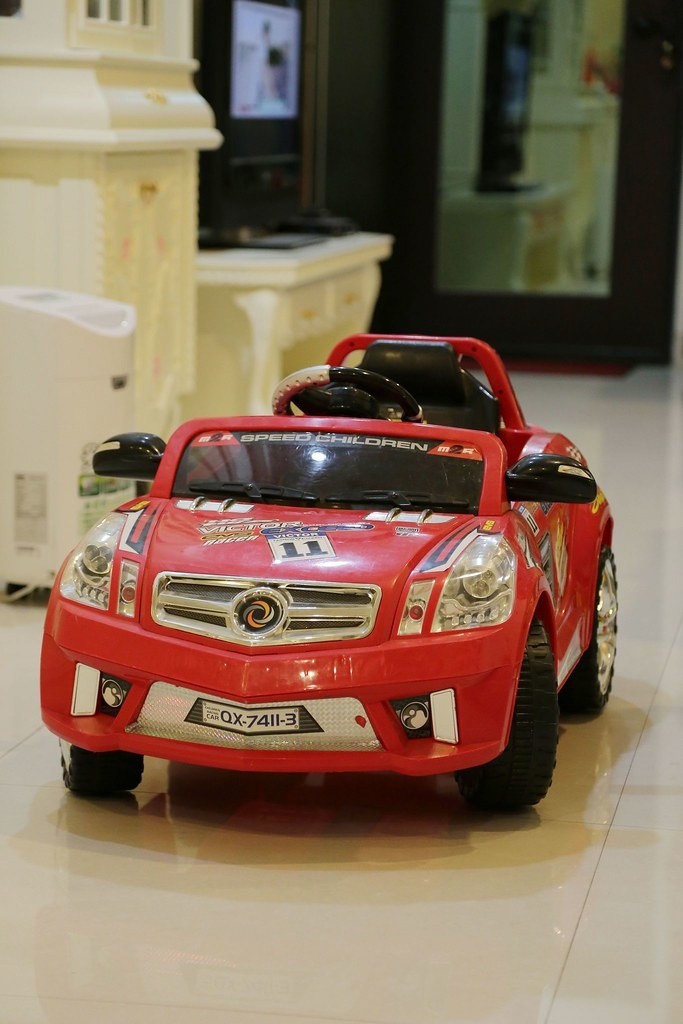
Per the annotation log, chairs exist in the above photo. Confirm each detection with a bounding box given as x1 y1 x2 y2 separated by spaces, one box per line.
325 340 499 435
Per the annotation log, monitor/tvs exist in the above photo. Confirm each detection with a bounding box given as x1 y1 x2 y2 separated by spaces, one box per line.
198 1 325 250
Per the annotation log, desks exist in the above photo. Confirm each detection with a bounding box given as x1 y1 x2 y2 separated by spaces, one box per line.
198 232 393 426
441 182 570 293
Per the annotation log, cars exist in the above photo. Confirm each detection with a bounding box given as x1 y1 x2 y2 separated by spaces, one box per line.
37 336 622 815
